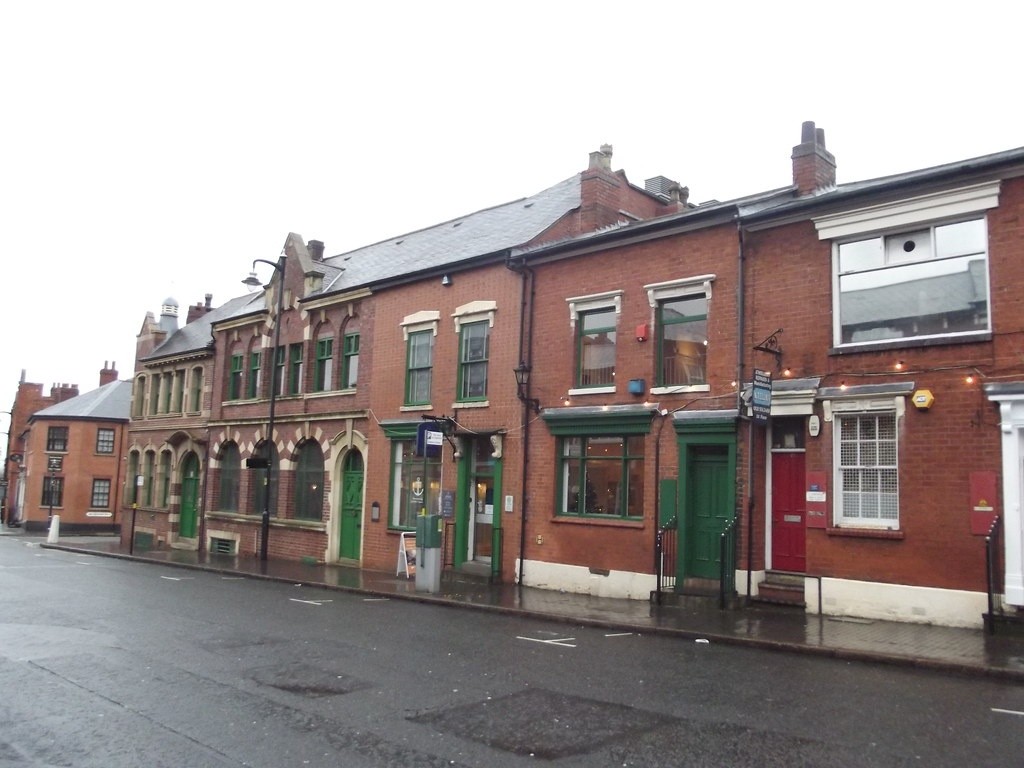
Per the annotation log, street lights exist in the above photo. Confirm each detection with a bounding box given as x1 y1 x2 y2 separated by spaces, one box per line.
240 256 288 562
